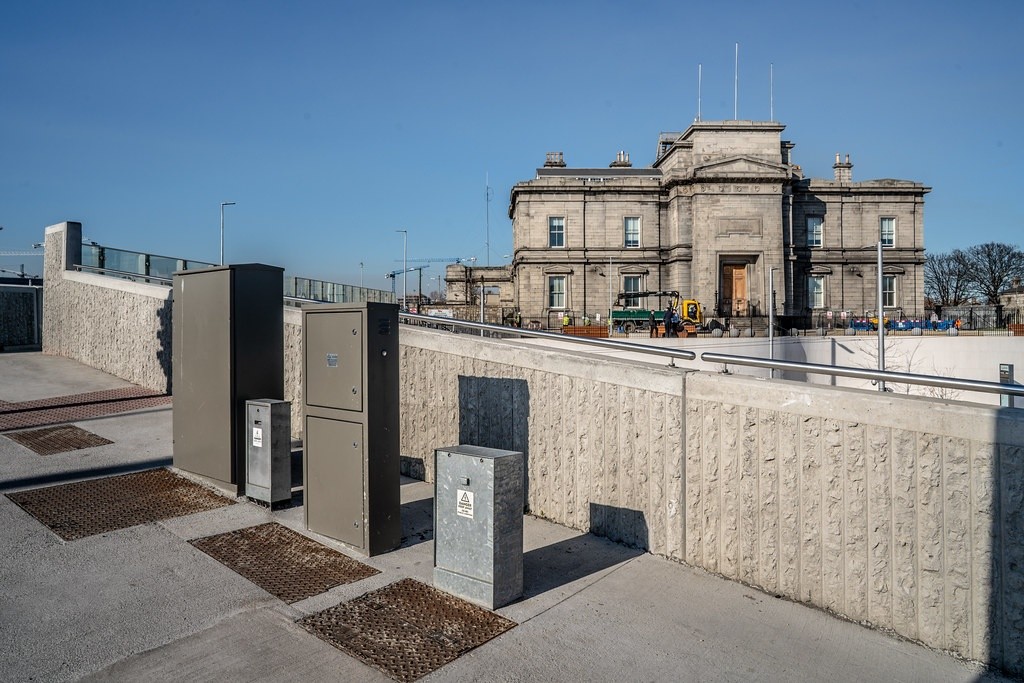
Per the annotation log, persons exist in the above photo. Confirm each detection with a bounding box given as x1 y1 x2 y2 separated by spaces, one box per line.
663 307 674 338
649 309 661 338
514 311 522 327
562 314 570 327
671 308 681 337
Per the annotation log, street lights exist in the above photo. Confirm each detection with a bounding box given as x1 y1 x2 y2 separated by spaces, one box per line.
395 229 407 311
220 201 236 265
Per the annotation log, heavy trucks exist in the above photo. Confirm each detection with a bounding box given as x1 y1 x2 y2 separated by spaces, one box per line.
608 290 706 335
